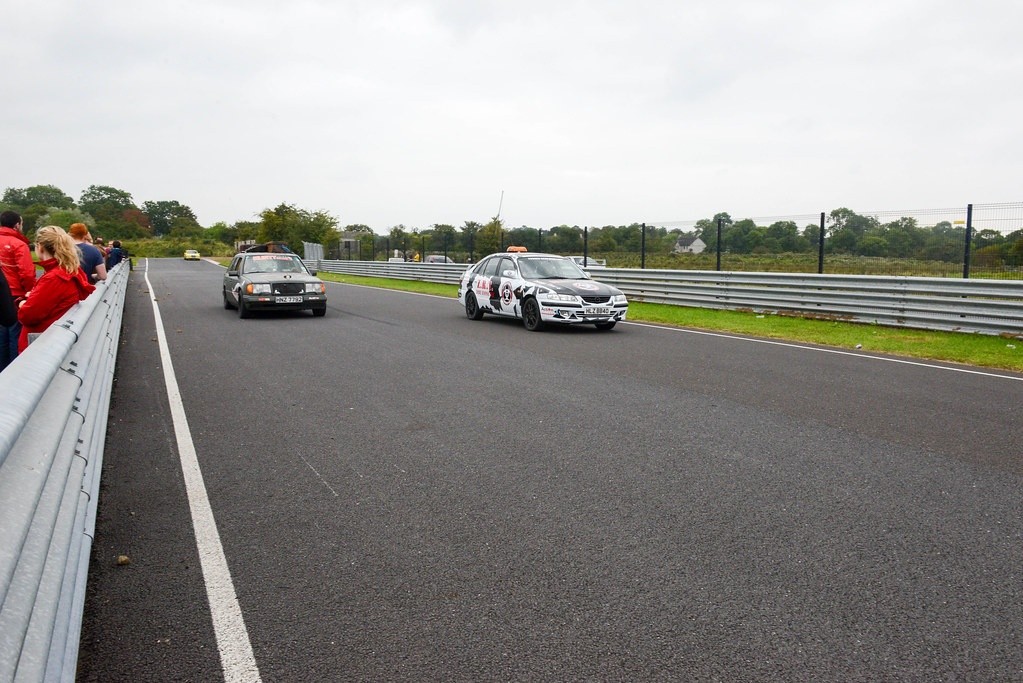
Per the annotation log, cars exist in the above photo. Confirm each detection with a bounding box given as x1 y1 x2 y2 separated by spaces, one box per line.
184 250 200 260
425 255 453 263
564 256 606 269
222 252 326 319
458 246 629 330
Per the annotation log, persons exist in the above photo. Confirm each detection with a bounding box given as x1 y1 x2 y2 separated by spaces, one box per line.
408 251 413 262
279 260 297 272
85 229 124 271
0 210 38 358
67 221 108 281
413 250 421 262
544 262 569 280
429 254 435 263
17 222 96 354
401 252 408 263
